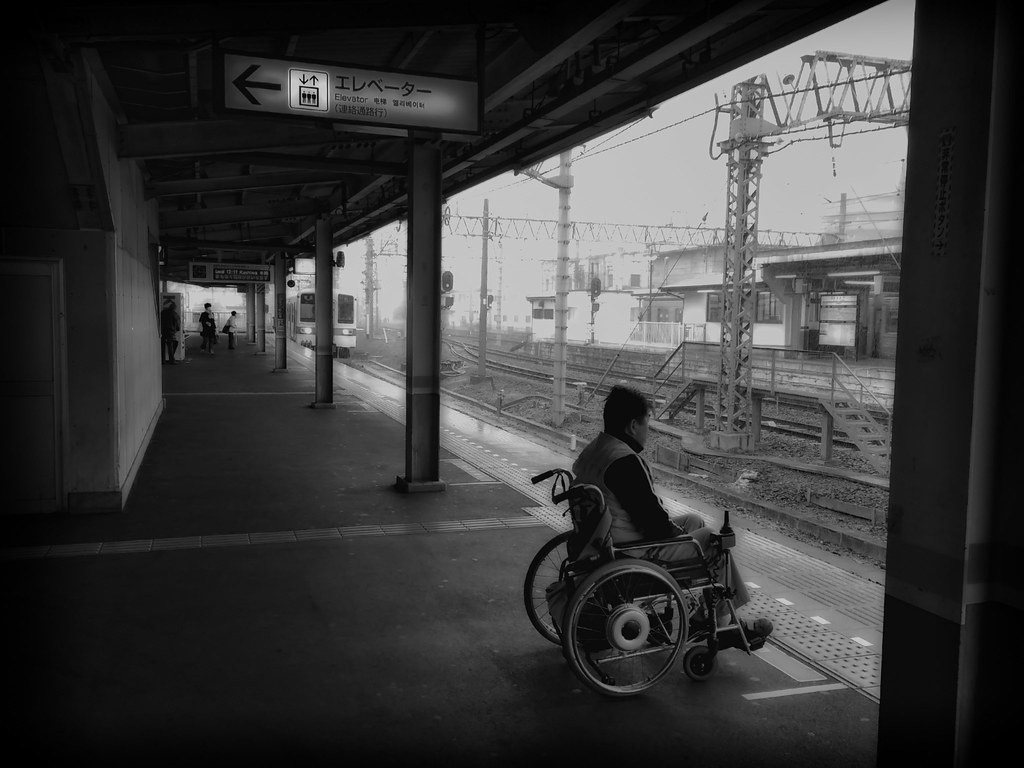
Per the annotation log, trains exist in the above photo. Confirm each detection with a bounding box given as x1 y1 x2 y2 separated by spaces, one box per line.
287 288 357 359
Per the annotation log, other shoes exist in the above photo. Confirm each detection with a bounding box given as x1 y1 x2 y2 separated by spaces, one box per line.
200 349 205 354
707 616 773 651
170 360 180 365
163 360 169 365
227 345 235 350
208 352 215 357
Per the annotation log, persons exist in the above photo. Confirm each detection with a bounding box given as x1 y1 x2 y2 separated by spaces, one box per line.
225 311 238 350
569 385 773 651
161 303 181 364
211 312 220 344
198 303 216 356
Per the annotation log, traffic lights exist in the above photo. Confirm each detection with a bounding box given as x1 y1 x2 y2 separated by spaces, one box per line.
441 271 453 293
591 277 602 298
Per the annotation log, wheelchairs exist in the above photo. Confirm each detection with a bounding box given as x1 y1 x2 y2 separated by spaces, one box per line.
524 468 766 699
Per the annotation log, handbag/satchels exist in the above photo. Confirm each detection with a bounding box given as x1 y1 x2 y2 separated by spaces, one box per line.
222 325 231 333
196 321 204 333
